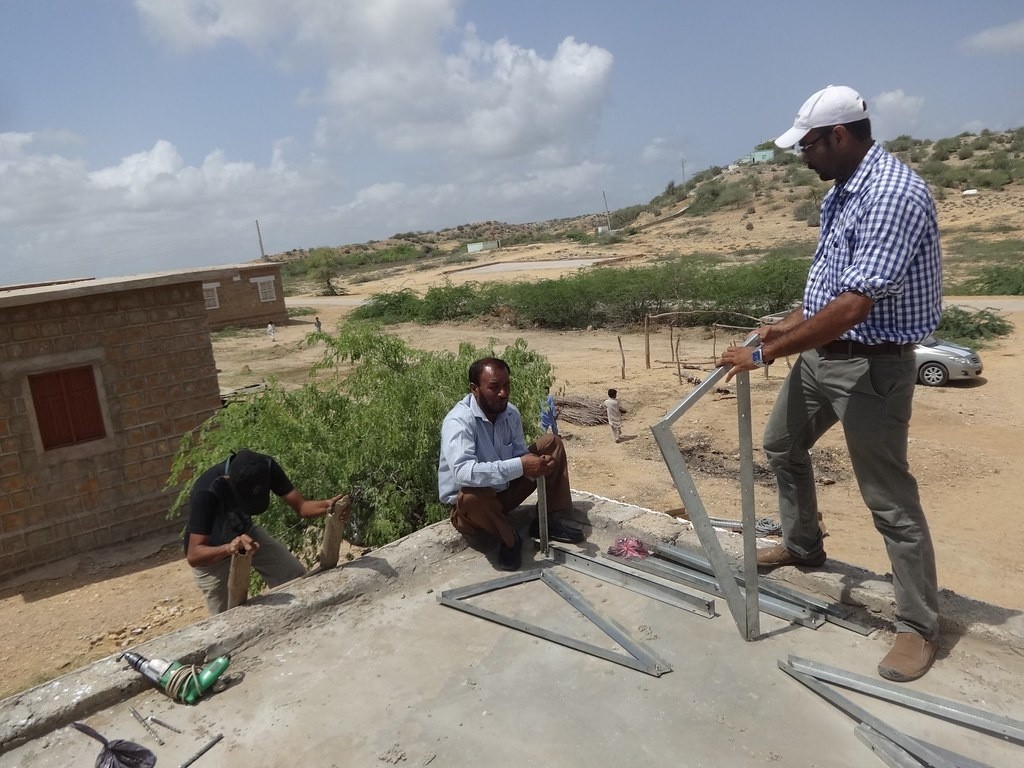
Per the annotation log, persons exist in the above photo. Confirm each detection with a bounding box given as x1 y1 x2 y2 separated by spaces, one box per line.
598 389 623 443
715 82 946 682
437 356 585 571
314 317 322 332
534 386 560 436
267 320 277 342
182 448 349 620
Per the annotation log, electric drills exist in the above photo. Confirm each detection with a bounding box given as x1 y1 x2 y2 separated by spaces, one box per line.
123 653 231 705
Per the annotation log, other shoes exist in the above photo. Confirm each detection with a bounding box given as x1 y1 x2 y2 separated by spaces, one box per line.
756 541 827 567
878 633 940 681
529 518 583 542
498 529 522 571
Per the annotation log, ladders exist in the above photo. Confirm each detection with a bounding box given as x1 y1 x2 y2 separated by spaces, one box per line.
226 495 350 610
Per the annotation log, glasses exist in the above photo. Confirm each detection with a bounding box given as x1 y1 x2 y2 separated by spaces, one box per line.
798 126 847 153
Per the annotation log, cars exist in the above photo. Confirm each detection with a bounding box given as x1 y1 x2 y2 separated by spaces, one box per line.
912 335 985 387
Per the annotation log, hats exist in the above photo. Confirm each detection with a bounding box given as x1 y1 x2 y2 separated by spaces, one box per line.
774 84 870 148
229 449 270 515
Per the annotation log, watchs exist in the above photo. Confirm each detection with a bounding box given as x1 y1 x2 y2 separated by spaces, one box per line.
752 344 775 368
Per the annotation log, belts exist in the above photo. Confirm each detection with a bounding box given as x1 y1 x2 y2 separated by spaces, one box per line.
821 340 912 356
